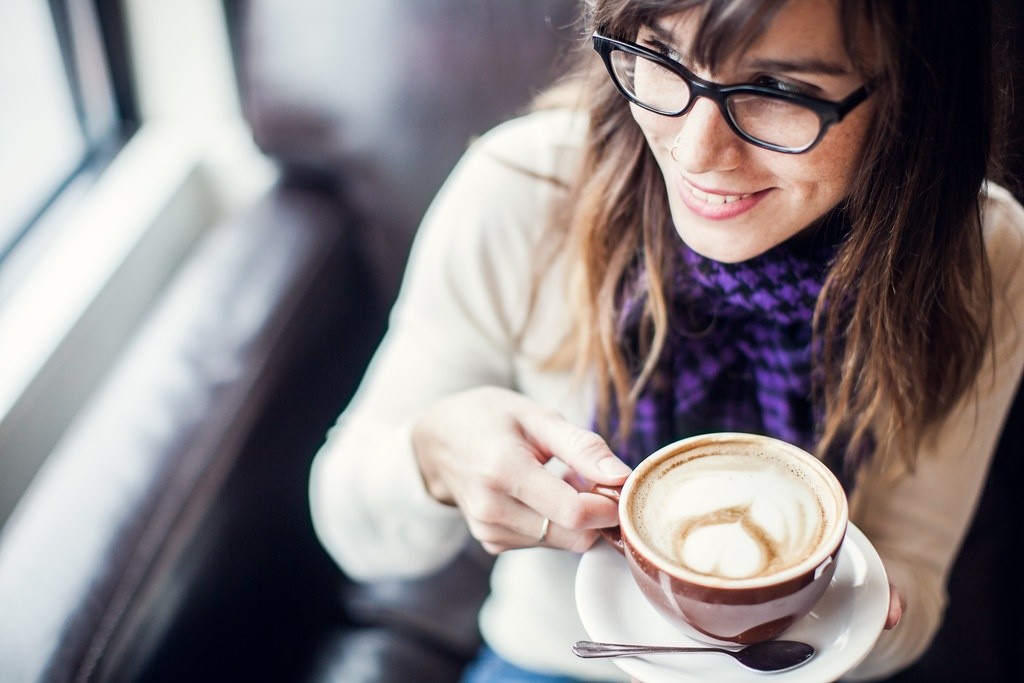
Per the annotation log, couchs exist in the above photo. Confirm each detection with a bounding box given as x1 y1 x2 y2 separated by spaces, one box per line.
234 1 1024 683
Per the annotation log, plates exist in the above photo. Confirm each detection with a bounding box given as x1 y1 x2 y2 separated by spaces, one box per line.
575 519 890 683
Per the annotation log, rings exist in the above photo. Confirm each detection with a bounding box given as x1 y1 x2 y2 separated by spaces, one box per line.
539 519 549 542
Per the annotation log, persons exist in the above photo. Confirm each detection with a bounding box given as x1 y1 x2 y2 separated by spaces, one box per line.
308 1 1024 682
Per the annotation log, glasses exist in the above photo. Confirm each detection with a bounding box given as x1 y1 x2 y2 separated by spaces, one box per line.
591 17 886 154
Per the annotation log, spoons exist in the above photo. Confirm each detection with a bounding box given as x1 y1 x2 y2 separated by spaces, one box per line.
571 640 817 675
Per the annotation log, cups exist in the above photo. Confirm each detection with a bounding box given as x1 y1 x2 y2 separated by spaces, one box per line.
591 431 848 649
